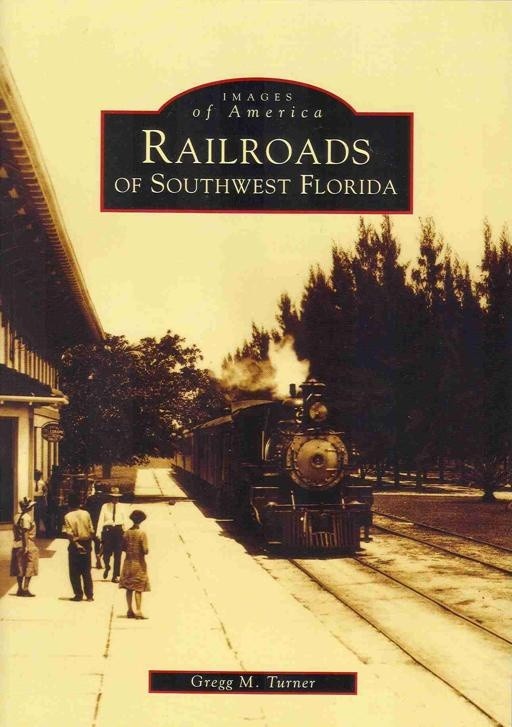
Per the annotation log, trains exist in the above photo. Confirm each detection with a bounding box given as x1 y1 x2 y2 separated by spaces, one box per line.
169 378 374 552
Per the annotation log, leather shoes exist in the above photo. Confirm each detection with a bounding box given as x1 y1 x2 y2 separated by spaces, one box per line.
17 588 95 602
103 566 121 584
127 609 149 620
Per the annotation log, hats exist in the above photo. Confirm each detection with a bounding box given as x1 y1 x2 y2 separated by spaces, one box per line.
18 497 39 511
108 487 123 498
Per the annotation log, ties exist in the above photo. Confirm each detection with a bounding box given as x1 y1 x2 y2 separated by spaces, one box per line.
112 503 116 523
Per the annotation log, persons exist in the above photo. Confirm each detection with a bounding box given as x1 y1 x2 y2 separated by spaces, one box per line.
117 508 152 620
94 485 131 583
10 496 40 597
34 469 53 538
85 481 110 569
61 492 101 601
